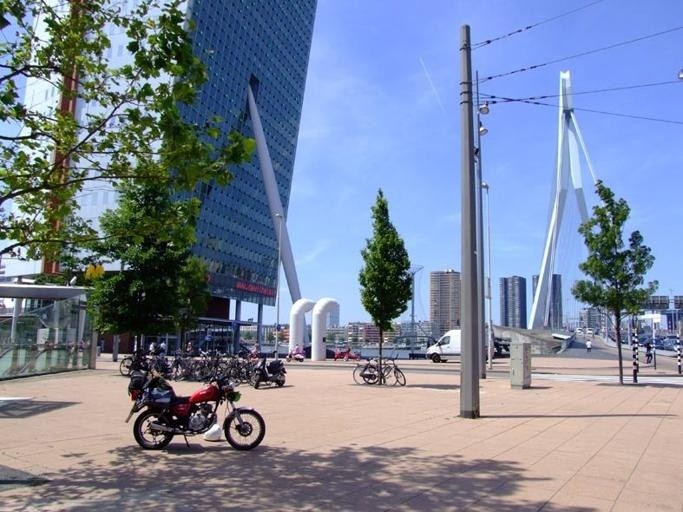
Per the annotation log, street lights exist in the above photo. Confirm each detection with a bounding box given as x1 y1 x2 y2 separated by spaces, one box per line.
482 182 492 369
274 214 282 360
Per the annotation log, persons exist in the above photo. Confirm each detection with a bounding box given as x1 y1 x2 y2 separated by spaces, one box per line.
291 344 299 358
100 337 106 353
159 340 167 356
150 340 156 353
645 343 652 364
585 339 593 352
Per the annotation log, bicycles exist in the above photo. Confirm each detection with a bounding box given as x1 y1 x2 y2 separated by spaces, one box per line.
119 349 259 386
353 354 406 387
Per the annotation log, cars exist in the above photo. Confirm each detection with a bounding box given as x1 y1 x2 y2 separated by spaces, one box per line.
637 335 683 351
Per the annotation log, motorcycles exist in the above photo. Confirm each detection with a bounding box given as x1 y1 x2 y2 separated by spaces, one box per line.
253 351 286 389
286 351 304 362
125 368 266 452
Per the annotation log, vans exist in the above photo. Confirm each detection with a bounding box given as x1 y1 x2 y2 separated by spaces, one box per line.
426 329 495 363
